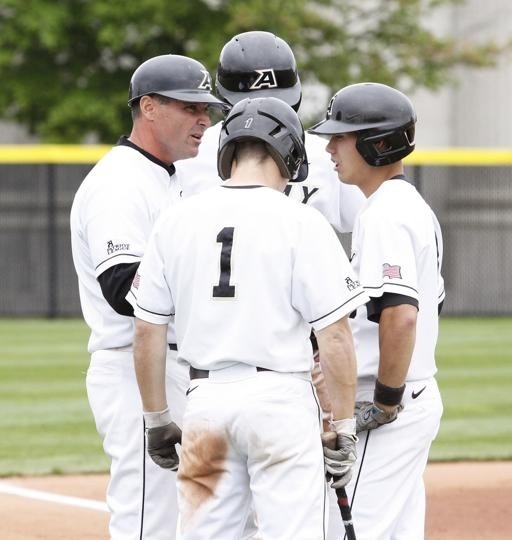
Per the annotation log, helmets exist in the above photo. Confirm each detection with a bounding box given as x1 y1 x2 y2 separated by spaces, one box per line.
216 31 301 107
307 82 417 166
217 97 308 182
128 53 224 106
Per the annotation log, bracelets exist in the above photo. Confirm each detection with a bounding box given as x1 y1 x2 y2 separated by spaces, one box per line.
375 381 408 406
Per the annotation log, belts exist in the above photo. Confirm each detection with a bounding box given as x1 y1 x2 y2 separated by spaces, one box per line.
190 365 273 379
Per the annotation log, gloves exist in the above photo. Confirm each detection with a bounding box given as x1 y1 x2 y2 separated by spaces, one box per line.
354 402 403 435
148 422 182 471
323 435 357 488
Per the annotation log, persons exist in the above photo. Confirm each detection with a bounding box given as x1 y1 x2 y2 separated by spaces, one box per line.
123 94 374 540
166 29 370 266
68 52 229 540
309 81 445 540
